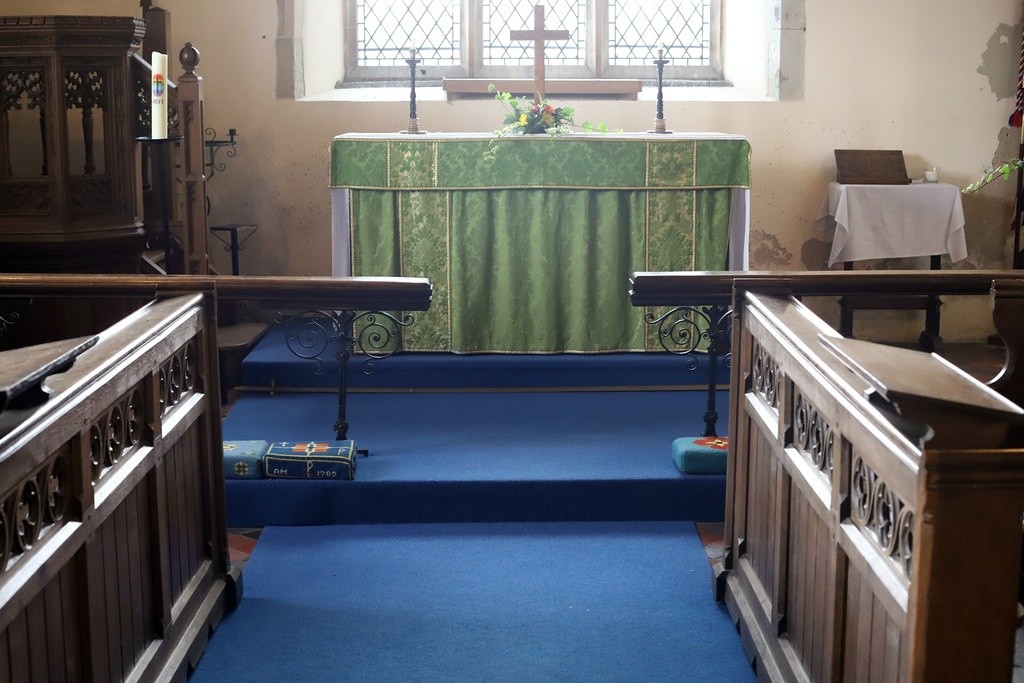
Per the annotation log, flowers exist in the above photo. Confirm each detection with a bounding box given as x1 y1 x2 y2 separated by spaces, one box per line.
479 78 628 167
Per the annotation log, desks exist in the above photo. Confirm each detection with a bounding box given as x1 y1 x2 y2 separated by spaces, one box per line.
325 130 757 354
824 179 964 352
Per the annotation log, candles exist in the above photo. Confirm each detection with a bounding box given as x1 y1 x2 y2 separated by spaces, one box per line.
657 35 664 51
408 36 416 51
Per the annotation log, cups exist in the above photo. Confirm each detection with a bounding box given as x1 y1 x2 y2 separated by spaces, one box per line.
925 167 940 183
